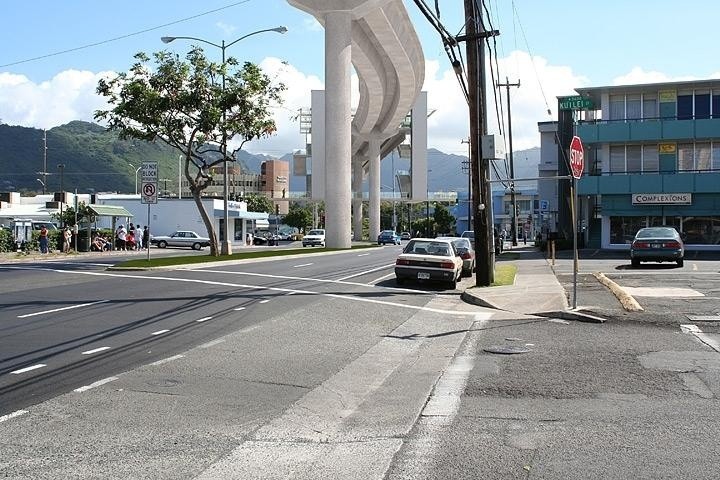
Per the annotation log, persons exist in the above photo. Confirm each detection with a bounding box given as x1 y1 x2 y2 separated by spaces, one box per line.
62 225 73 254
40 224 50 253
523 231 528 245
501 228 507 243
92 222 149 252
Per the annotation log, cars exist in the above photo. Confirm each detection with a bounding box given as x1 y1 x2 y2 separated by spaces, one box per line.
629 227 686 268
399 232 411 240
461 230 475 251
80 227 101 230
378 229 401 245
235 231 267 246
396 237 464 287
303 229 326 247
150 229 210 251
0 219 57 232
436 235 475 278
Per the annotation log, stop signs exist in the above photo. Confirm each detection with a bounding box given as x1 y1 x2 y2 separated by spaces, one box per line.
569 137 586 179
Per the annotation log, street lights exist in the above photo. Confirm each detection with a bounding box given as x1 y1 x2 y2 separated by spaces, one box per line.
129 164 141 194
36 177 45 196
160 25 289 257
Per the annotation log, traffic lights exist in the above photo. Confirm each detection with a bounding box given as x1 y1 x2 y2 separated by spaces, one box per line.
456 199 458 205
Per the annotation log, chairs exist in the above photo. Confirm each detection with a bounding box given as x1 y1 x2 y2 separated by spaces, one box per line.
438 248 447 253
415 248 425 252
